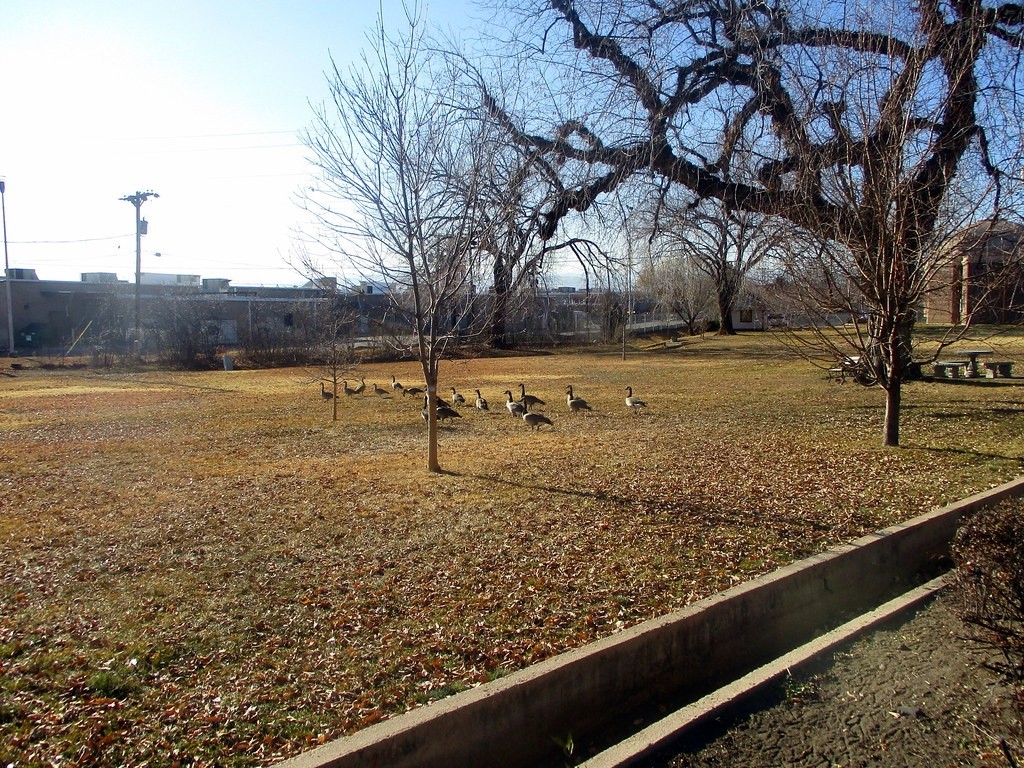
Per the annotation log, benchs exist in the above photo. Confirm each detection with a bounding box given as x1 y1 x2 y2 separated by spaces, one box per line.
826 356 861 385
933 360 1015 378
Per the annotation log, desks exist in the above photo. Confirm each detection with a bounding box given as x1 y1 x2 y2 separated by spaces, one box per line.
956 350 994 378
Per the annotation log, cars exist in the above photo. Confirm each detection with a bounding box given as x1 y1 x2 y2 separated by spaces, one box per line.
767 314 787 327
858 316 867 323
695 314 704 319
669 314 677 321
698 311 703 314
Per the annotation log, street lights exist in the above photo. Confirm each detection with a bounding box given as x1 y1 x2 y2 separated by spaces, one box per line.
118 189 160 361
0 175 19 358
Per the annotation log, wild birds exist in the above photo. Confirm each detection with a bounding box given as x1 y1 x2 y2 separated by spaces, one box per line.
343 376 366 397
565 385 593 416
319 382 340 404
391 375 403 391
421 387 466 422
403 387 423 399
504 384 553 431
475 390 489 414
624 386 647 414
372 384 390 398
